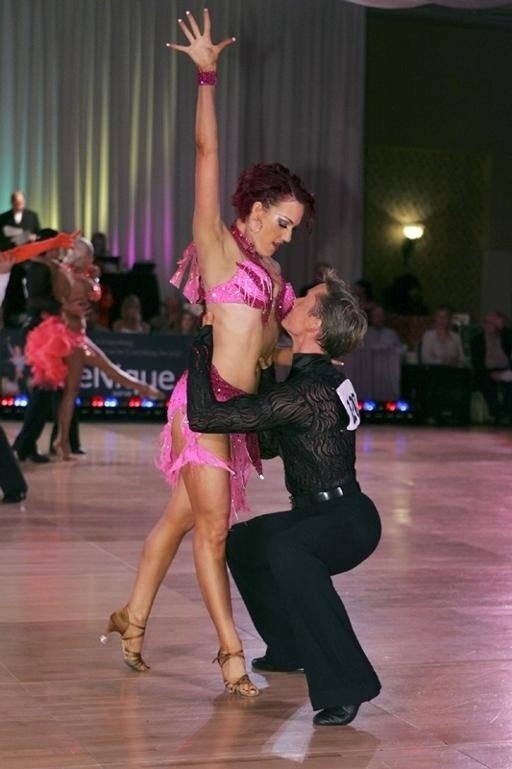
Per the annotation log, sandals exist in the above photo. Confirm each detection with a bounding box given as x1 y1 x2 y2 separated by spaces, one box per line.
141 384 160 399
52 442 77 461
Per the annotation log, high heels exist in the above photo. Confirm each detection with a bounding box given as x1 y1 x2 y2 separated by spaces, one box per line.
98 610 150 671
211 648 259 697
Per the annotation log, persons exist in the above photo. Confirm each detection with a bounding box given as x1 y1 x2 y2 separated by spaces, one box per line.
1 189 159 504
183 265 384 729
299 260 512 428
153 293 197 334
97 2 348 699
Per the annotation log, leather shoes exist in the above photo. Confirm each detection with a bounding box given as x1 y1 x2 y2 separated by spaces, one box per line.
0 493 27 503
16 450 50 464
253 656 302 671
313 702 361 725
72 447 86 454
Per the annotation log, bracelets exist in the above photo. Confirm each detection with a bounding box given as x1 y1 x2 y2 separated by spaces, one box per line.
195 69 218 86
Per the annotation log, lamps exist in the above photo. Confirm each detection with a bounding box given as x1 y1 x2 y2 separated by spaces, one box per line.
400 224 425 265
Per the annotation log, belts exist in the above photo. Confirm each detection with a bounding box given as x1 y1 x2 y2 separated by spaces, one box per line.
289 483 362 506
